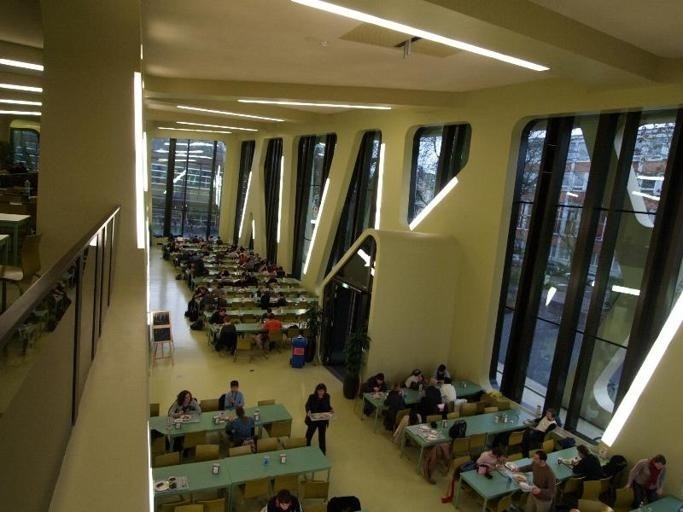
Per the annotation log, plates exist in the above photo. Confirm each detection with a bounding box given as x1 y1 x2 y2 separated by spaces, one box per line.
494 459 528 488
563 455 581 466
154 476 177 491
165 415 192 430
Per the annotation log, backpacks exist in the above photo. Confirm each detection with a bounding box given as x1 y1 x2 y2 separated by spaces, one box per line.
449 420 466 438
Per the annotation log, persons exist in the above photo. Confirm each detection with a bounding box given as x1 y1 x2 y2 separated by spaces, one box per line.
260 489 303 512
163 233 309 368
225 381 244 441
305 384 332 456
225 407 254 442
168 390 201 458
359 365 667 512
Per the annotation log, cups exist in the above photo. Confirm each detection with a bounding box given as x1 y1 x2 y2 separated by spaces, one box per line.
418 383 422 392
441 419 447 428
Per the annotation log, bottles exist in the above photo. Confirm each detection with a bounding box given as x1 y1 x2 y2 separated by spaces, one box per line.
493 411 514 424
262 454 269 466
23 180 30 199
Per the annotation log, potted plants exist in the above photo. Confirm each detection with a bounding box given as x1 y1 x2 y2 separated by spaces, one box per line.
303 296 325 363
341 328 372 400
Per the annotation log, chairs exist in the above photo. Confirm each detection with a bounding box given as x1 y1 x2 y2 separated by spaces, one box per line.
363 380 682 512
149 396 334 511
0 166 38 314
162 236 317 362
